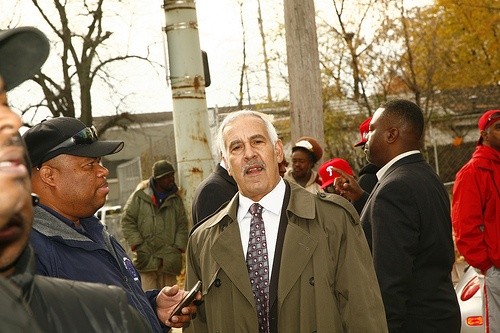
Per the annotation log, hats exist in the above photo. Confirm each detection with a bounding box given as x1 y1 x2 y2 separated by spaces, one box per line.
317 158 356 189
153 161 175 178
292 137 323 161
0 26 51 91
22 117 125 168
354 117 373 147
477 109 500 145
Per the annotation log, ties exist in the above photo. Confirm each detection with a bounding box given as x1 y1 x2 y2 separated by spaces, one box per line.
246 203 270 333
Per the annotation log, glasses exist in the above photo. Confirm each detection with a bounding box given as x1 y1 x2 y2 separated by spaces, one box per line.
37 124 99 171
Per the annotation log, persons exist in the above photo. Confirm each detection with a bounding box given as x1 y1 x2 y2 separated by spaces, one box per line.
191 117 373 226
183 110 388 332
452 110 499 332
0 25 202 333
330 98 462 332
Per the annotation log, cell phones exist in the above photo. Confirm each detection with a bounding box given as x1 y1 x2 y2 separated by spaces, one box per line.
168 280 202 322
